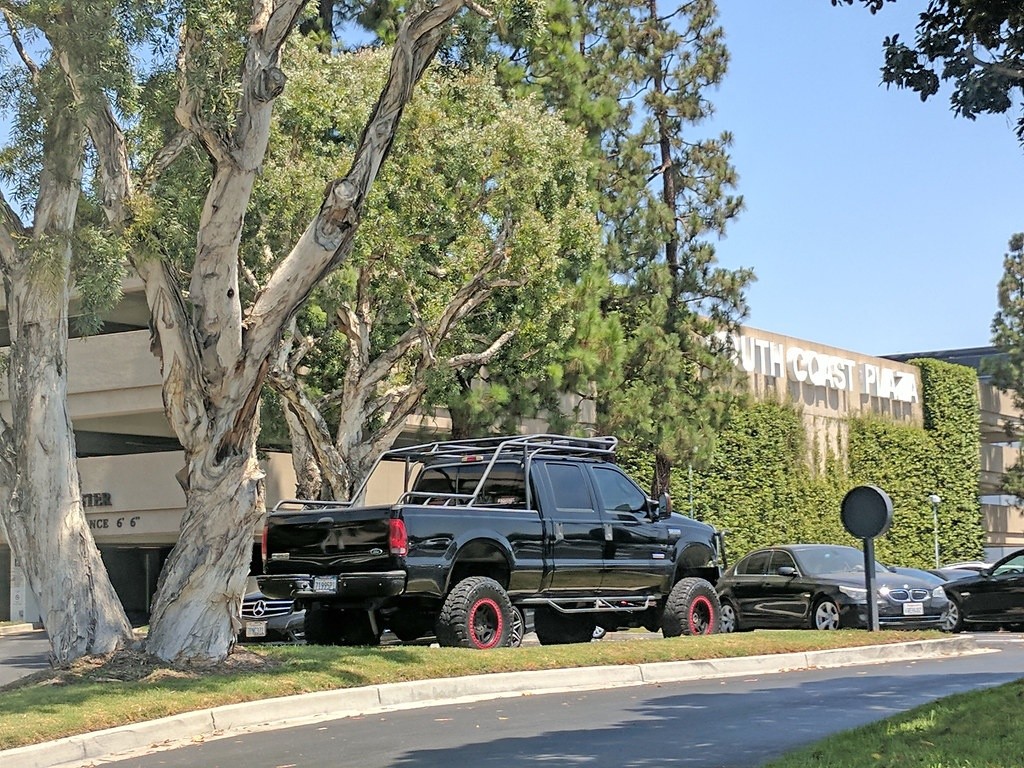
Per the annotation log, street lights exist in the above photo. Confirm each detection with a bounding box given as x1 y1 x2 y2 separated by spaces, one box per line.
929 492 943 571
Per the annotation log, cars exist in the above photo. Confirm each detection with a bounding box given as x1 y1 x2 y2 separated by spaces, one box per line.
713 543 946 630
932 549 1024 634
888 559 1022 590
238 575 607 640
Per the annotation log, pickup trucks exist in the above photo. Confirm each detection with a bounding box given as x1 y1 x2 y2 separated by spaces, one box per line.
254 433 728 650
